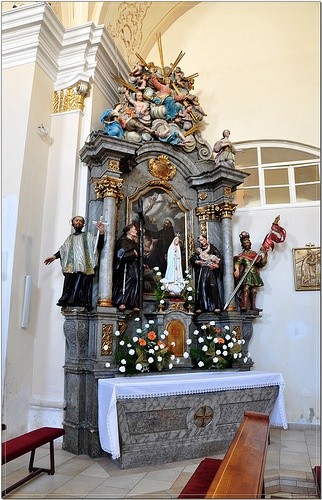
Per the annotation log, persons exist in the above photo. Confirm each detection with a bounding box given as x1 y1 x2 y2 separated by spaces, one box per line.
189 234 224 314
165 236 184 284
233 231 269 312
114 225 141 312
101 62 237 167
44 216 106 313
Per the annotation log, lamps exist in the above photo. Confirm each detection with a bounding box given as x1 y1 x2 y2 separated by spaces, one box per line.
22 275 33 328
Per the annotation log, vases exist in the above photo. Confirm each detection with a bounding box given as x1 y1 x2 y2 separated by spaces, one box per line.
158 304 165 312
187 304 194 314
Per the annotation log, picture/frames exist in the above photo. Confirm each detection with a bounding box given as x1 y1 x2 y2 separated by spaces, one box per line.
292 248 321 292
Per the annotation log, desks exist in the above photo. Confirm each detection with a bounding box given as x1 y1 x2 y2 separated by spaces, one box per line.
98 371 288 470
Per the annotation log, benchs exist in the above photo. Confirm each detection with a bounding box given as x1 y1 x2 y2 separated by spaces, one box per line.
1 427 67 499
176 410 271 499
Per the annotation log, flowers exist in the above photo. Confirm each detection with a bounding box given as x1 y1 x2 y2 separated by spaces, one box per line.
153 266 196 305
114 318 180 376
183 321 250 370
